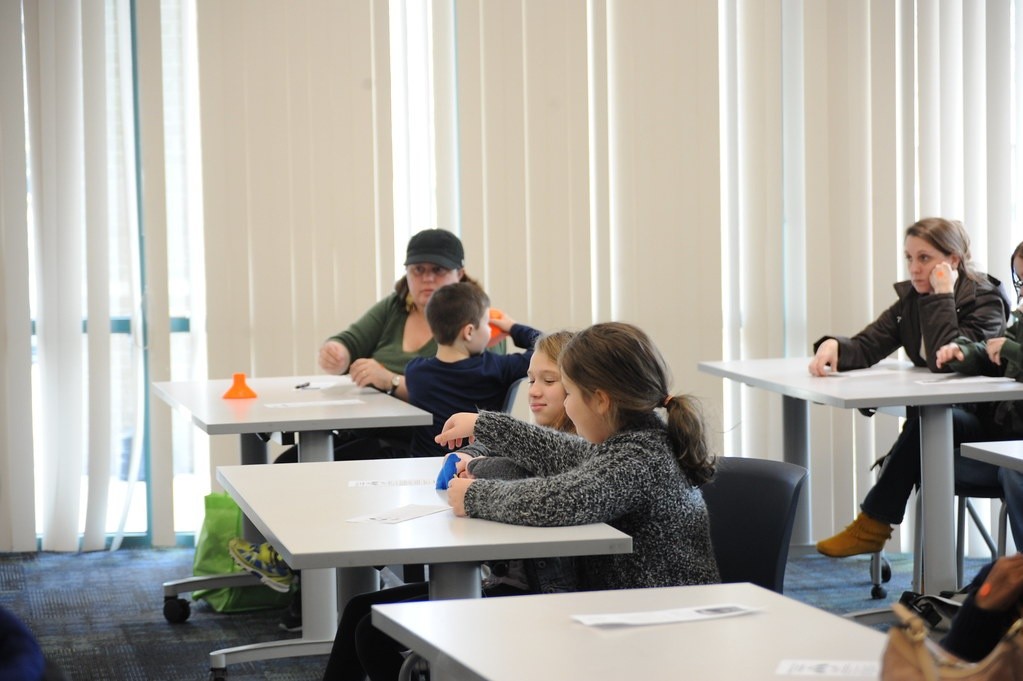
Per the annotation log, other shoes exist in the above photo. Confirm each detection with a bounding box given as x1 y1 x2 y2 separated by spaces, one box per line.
817 513 894 557
278 594 301 631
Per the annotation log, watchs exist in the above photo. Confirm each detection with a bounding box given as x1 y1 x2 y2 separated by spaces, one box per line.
387 373 400 399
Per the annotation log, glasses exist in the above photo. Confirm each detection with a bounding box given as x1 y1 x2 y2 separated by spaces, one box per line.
1013 274 1023 291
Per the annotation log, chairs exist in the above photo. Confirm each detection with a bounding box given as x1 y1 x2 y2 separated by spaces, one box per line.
684 453 801 596
870 465 1010 597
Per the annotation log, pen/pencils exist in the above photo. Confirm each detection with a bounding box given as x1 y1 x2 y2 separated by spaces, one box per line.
295 382 310 389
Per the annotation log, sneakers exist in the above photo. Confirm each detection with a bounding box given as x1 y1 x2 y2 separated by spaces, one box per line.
229 538 299 593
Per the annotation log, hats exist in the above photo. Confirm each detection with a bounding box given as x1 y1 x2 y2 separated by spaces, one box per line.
404 229 464 269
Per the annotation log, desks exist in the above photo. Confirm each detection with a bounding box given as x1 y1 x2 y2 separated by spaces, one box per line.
372 581 892 680
150 374 433 619
697 357 1023 598
209 455 634 666
959 435 1023 480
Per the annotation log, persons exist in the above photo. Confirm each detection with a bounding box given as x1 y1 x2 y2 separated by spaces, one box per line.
228 228 722 681
809 217 1023 681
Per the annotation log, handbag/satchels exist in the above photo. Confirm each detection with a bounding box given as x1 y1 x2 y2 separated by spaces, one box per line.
192 490 277 612
879 602 1023 680
